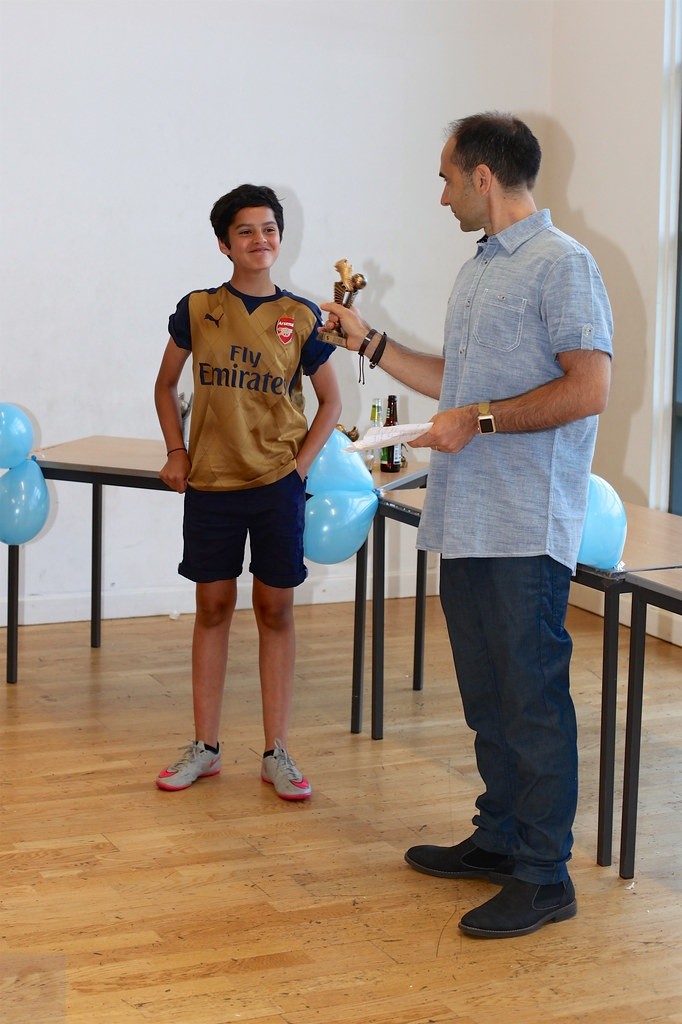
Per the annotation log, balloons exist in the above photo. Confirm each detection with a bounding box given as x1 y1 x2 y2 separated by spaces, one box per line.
0 403 33 468
577 474 627 570
0 456 49 545
301 428 375 496
304 490 381 565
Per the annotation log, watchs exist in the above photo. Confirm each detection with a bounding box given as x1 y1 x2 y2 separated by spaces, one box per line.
477 402 496 435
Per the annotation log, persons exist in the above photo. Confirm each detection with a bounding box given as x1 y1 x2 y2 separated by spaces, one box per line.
155 184 343 800
317 113 614 938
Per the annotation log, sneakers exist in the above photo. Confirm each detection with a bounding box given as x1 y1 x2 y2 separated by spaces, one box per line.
458 882 579 937
261 740 313 800
403 838 512 883
157 739 223 792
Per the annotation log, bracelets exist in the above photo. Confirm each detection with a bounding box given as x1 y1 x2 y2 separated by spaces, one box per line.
358 329 378 384
167 448 187 456
369 332 387 369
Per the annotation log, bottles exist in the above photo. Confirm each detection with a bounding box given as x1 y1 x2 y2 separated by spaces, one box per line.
369 399 382 472
381 395 401 473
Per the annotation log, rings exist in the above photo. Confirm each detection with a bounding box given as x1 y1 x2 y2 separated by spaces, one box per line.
435 446 438 451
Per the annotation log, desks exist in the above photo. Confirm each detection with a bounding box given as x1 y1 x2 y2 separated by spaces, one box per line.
7 434 429 734
618 567 682 880
372 486 682 867
